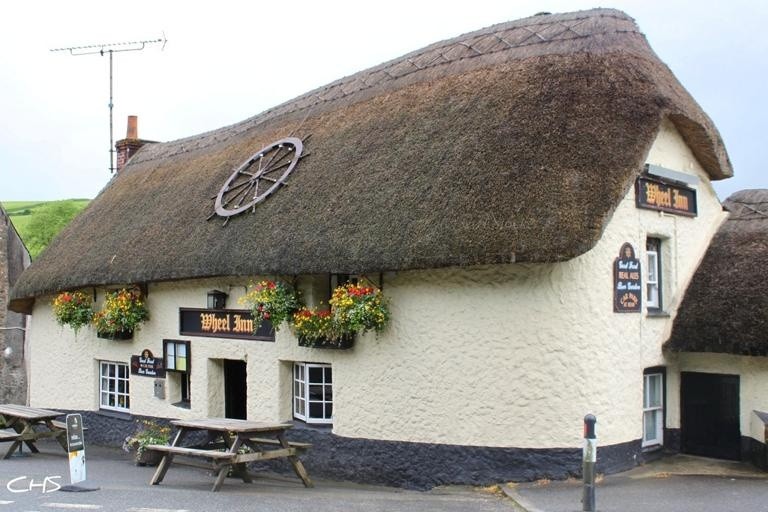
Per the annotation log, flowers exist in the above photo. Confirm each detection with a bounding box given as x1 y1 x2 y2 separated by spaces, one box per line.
236 275 390 349
120 418 171 466
52 278 149 343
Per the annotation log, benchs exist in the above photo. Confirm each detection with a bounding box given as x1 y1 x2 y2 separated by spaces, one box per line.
0 430 22 440
145 445 237 462
230 435 313 450
39 420 88 432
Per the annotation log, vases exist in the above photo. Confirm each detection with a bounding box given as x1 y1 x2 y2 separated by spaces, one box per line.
136 446 169 466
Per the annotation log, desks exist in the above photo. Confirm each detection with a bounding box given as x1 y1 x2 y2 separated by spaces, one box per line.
0 403 68 459
150 417 314 493
